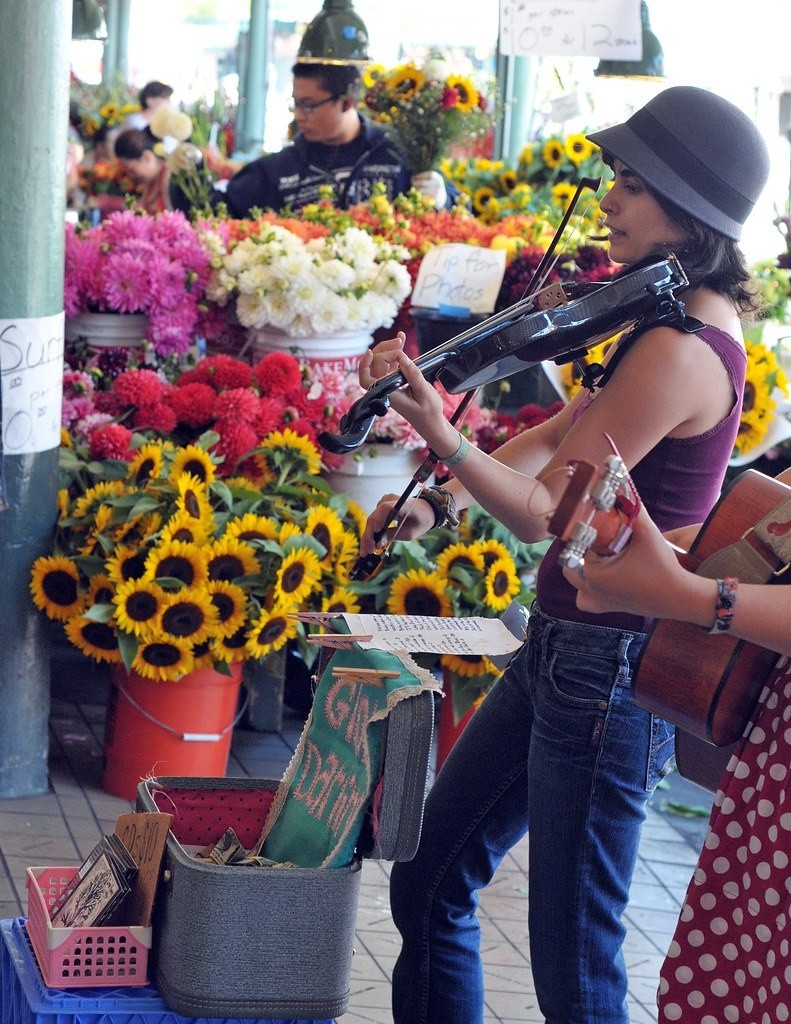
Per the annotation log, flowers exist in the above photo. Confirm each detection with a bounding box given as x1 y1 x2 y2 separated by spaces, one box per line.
60 345 352 478
77 159 147 197
63 195 209 359
208 223 410 333
478 402 564 455
360 64 494 172
30 433 364 680
561 262 789 458
369 526 525 726
214 134 613 270
316 357 486 478
81 92 137 138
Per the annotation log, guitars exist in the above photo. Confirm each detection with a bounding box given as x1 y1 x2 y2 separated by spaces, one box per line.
544 455 791 796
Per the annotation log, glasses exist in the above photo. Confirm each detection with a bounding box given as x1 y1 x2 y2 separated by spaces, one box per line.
288 96 340 116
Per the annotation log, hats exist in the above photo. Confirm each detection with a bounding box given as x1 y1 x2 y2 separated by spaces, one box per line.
586 86 771 242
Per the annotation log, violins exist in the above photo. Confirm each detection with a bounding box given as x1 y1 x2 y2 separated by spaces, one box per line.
314 242 707 455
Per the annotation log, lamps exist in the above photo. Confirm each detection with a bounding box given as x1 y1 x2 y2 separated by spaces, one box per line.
71 1 108 41
299 0 371 64
596 0 663 81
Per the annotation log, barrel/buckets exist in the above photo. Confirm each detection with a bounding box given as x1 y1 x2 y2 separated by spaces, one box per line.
436 670 476 783
322 442 436 522
207 309 376 392
65 313 152 370
103 654 246 800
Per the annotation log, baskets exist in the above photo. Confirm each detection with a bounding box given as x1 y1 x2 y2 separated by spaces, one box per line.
24 865 154 990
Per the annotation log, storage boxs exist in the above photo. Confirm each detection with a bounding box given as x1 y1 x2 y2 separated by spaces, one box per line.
0 918 334 1024
24 866 146 989
138 615 434 1020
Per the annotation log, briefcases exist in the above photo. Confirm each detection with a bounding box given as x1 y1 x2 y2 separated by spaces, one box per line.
133 616 434 1019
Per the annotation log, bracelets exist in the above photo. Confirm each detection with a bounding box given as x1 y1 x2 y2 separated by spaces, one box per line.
710 577 739 634
412 485 460 530
440 432 469 467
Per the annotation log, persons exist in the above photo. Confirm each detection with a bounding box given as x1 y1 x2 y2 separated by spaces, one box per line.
562 467 791 1024
358 91 773 1024
66 60 473 218
